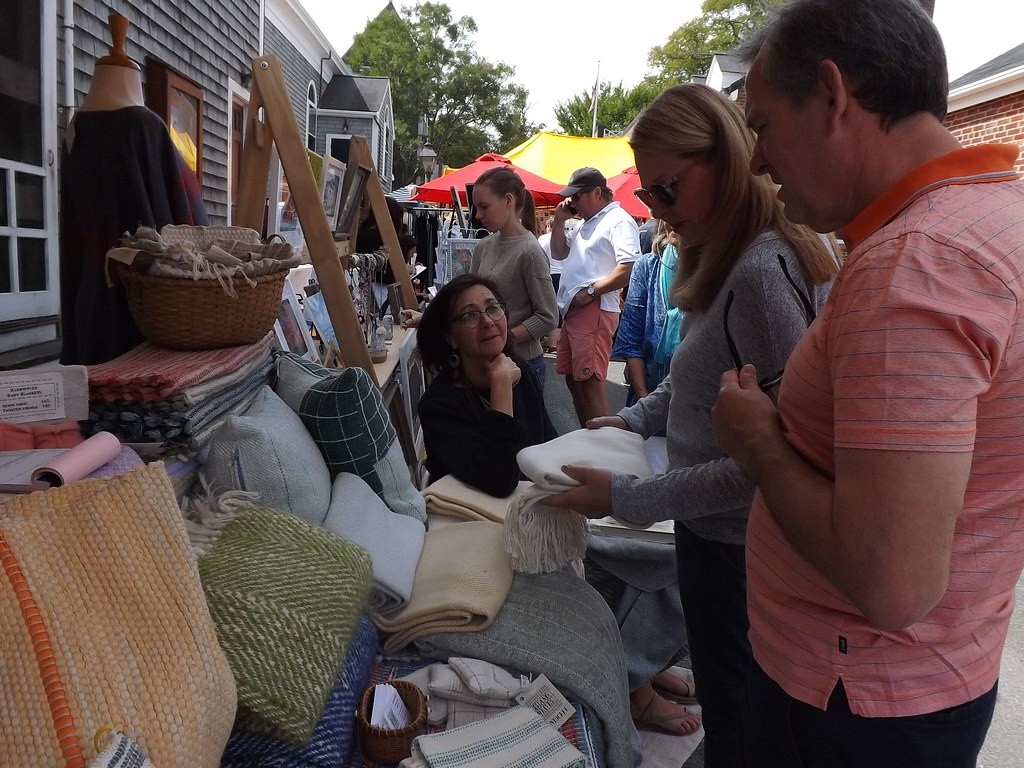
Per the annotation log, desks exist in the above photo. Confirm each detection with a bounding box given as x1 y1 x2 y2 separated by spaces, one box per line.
308 317 415 393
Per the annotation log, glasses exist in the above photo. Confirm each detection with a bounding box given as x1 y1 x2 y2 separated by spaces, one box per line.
447 302 507 329
635 153 707 210
568 191 587 202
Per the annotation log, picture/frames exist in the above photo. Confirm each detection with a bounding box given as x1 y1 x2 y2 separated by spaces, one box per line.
383 381 421 491
387 282 406 325
144 55 204 189
333 161 371 238
266 143 303 252
320 153 345 231
272 278 318 362
399 328 426 461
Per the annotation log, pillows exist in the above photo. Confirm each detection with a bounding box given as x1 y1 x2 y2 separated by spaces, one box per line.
202 384 333 526
273 349 427 524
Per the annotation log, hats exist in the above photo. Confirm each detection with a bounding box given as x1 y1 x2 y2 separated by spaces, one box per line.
556 170 606 197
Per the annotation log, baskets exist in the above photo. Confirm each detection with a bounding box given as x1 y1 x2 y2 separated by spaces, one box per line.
357 680 429 764
110 233 291 350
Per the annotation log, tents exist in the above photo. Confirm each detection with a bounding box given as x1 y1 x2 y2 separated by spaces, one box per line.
504 132 635 185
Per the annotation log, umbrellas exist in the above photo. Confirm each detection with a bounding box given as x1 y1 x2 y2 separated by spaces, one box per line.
410 154 566 207
384 183 418 203
606 166 650 218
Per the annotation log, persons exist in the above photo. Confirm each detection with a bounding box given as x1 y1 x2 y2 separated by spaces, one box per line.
538 84 839 768
417 274 705 734
550 167 643 429
538 217 563 358
59 51 208 365
399 166 560 390
608 219 683 437
710 0 1023 768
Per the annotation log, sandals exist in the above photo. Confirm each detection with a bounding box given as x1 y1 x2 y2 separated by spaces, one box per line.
650 666 700 704
632 691 701 736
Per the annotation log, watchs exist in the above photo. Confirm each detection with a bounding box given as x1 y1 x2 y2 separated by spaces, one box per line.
587 284 596 298
